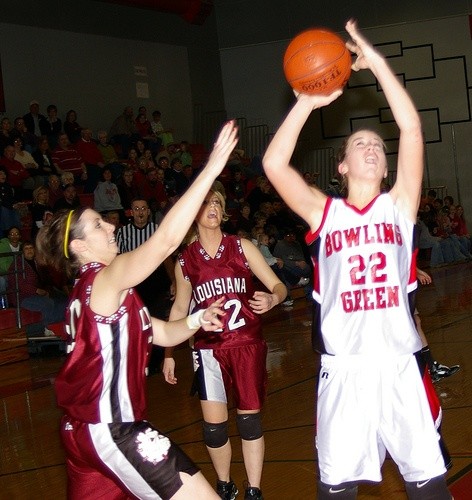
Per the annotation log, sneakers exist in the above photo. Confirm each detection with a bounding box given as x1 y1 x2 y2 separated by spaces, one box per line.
216 478 238 500
431 360 460 384
244 485 264 500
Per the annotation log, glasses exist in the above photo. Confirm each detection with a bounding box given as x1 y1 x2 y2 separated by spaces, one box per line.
132 207 148 210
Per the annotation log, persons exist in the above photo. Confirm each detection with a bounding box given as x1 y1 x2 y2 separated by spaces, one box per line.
36 118 239 500
177 134 347 306
382 180 471 381
116 197 171 376
263 19 454 500
0 99 177 337
162 181 287 500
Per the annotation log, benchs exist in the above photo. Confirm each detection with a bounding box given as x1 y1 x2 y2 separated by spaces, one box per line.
2 143 308 329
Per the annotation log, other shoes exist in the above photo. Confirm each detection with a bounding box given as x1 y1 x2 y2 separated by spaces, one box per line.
297 276 310 286
282 301 293 306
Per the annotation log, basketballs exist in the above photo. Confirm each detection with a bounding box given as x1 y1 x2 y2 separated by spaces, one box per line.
282 30 352 94
